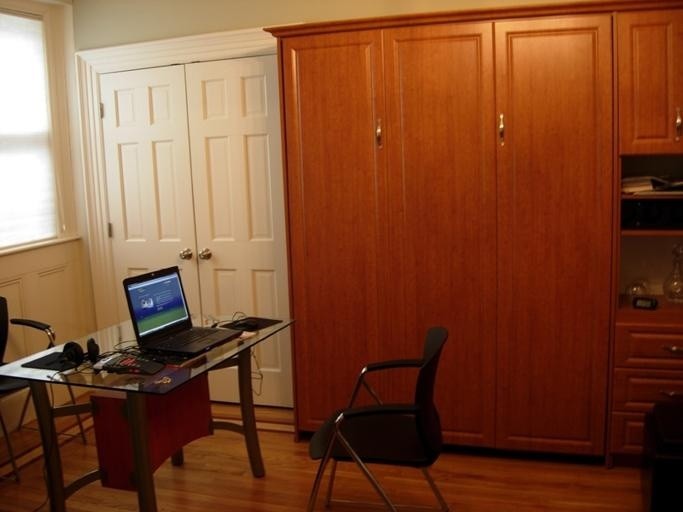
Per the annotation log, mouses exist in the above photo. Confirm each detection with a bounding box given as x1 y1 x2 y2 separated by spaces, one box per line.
232 318 259 329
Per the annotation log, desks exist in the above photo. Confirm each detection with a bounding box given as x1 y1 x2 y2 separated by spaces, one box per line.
0 313 294 512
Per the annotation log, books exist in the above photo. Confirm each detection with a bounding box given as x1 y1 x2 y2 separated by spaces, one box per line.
621 175 683 195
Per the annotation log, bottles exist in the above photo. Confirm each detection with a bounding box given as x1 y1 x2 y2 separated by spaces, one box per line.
662 256 683 304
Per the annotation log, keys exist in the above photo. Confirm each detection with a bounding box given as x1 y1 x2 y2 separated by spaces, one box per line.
152 375 172 384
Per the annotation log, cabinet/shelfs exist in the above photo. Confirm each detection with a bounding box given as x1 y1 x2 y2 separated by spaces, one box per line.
261 3 614 466
617 324 682 468
618 159 683 236
610 1 681 155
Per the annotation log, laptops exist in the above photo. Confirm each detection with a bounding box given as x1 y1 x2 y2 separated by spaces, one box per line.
123 266 243 357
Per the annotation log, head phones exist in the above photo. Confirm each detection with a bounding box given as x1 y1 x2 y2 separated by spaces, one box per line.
58 338 100 367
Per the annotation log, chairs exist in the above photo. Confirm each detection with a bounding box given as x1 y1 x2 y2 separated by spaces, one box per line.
0 294 87 486
303 326 452 510
